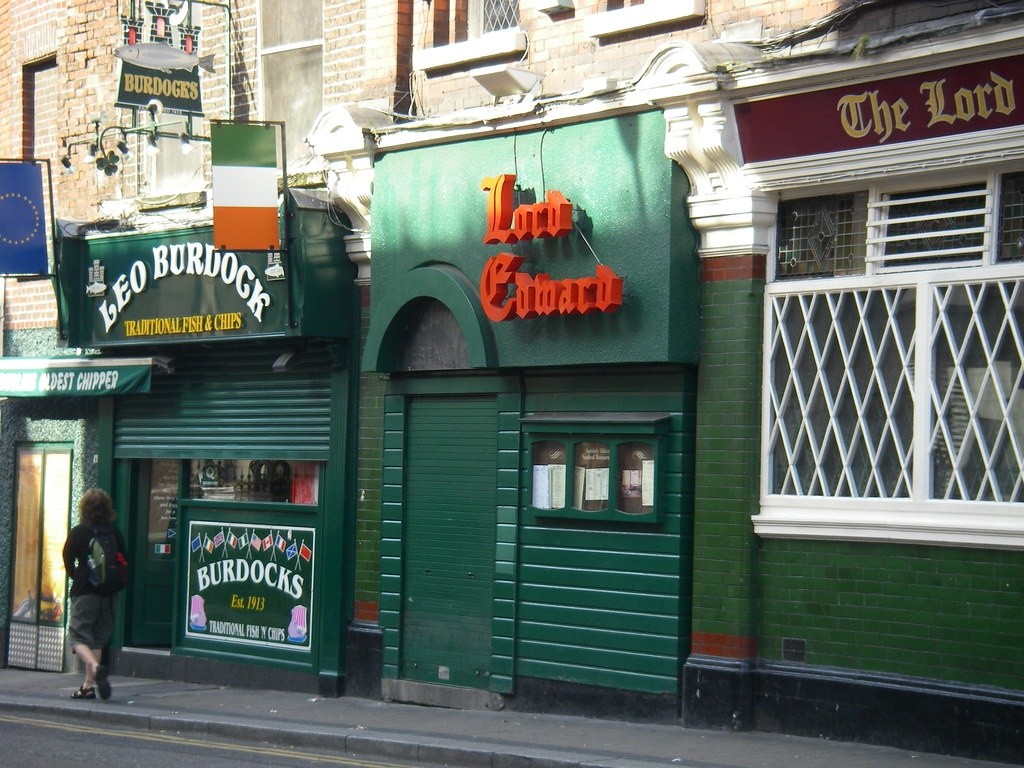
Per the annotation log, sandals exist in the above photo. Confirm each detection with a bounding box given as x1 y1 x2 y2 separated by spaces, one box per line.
70 684 96 699
93 664 112 700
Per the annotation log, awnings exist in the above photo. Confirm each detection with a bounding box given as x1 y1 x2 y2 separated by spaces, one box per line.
0 354 178 396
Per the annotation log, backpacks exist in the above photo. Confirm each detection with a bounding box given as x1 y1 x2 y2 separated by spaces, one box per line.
78 533 129 596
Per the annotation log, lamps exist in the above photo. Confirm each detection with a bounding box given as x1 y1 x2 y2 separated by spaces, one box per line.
473 67 543 107
61 100 193 176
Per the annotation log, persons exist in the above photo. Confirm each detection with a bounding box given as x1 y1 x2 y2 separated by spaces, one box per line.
62 487 130 701
